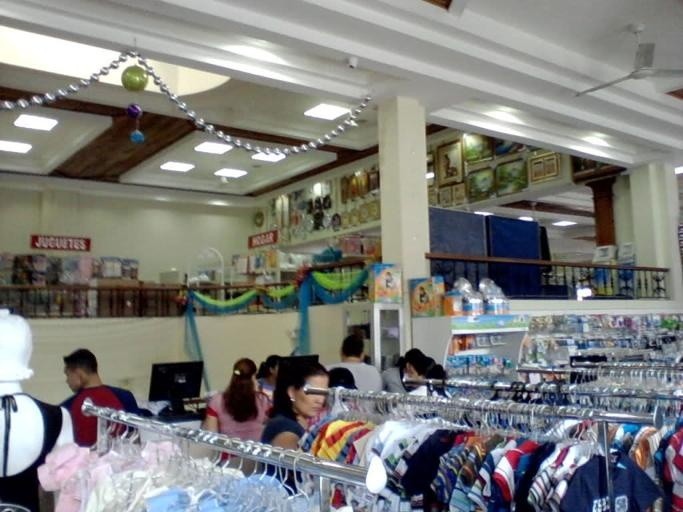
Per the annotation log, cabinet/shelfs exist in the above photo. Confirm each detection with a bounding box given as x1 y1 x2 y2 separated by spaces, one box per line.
341 299 403 371
410 305 683 376
428 203 543 300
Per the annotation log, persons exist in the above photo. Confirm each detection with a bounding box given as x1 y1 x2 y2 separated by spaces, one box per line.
202 334 447 493
55 348 139 446
0 310 74 512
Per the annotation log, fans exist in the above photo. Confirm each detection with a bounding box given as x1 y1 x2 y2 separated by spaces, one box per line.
574 21 683 97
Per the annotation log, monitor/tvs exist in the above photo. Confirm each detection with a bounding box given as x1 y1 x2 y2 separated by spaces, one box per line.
148 361 205 421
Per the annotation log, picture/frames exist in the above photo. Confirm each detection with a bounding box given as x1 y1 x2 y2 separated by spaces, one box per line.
424 129 562 205
